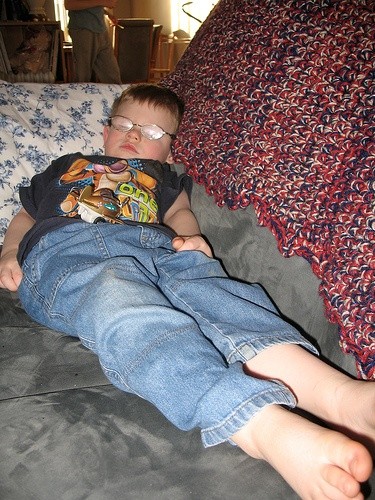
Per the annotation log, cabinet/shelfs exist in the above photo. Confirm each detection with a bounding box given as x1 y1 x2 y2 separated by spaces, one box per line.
0 20 68 83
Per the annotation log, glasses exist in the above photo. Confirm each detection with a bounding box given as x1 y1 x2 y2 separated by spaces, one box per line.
108 115 176 140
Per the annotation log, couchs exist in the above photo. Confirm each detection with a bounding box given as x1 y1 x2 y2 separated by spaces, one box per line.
0 0 375 500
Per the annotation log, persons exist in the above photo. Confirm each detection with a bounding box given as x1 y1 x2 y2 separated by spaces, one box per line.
0 84 375 500
65 0 122 84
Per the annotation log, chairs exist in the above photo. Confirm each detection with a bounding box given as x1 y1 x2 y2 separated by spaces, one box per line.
58 17 194 86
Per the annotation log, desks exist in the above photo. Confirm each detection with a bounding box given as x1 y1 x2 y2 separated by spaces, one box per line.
158 35 194 84
